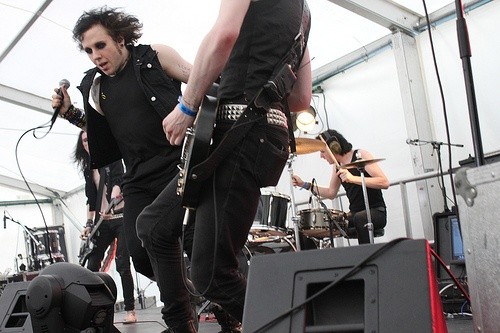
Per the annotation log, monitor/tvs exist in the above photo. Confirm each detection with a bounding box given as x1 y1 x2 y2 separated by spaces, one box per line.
448 215 465 264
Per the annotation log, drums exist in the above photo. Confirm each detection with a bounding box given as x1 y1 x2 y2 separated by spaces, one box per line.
249 191 291 236
297 208 348 240
28 229 64 262
246 230 317 263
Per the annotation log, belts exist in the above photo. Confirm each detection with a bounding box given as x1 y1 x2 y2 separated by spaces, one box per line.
216 102 290 128
104 213 123 220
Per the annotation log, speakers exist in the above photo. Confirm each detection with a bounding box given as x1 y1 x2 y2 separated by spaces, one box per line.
431 210 471 304
241 239 449 333
0 282 33 333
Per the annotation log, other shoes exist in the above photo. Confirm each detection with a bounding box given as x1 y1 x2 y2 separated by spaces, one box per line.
123 311 137 322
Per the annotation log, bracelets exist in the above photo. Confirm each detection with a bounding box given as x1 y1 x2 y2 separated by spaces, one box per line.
62 104 86 127
302 182 311 190
179 102 197 117
86 219 94 227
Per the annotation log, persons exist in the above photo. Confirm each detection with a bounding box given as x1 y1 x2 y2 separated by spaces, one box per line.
292 129 390 249
52 7 199 333
73 129 137 324
162 0 312 323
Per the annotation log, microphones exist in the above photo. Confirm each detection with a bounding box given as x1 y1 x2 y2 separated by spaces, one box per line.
308 178 315 204
50 78 70 127
4 211 6 228
406 138 418 144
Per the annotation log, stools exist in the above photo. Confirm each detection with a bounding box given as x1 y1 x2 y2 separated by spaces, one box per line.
345 227 383 238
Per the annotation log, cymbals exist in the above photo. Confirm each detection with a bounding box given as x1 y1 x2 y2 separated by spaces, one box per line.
337 157 385 169
288 137 326 154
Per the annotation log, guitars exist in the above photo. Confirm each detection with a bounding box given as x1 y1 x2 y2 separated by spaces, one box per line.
78 195 124 266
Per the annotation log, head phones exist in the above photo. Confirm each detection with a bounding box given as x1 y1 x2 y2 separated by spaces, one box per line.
324 130 342 154
